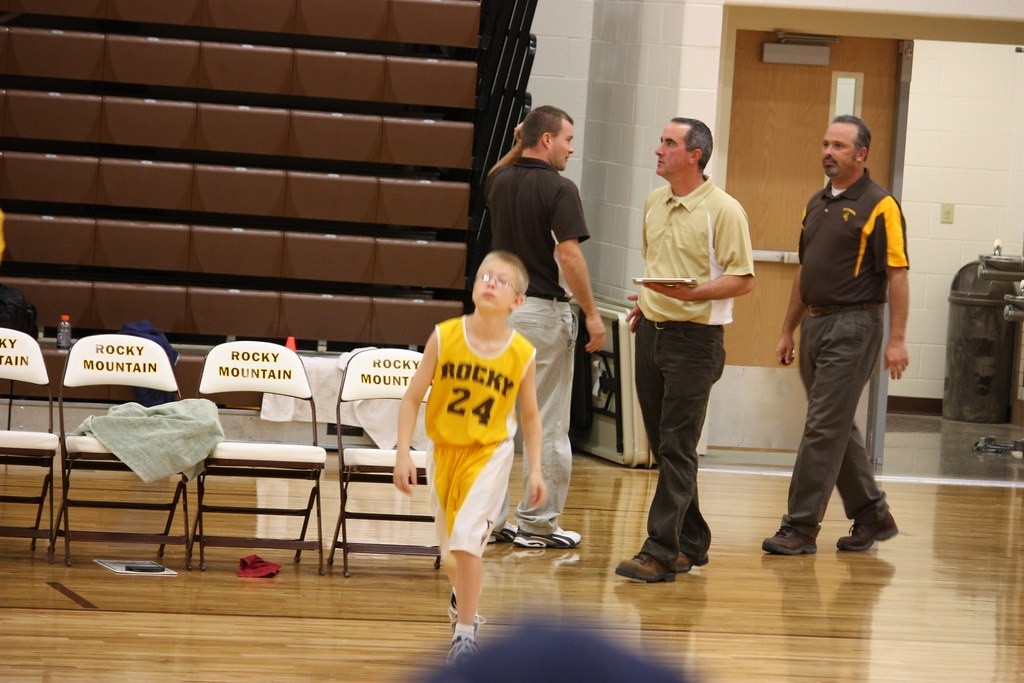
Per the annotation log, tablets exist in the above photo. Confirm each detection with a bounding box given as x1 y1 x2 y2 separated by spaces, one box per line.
633 278 698 285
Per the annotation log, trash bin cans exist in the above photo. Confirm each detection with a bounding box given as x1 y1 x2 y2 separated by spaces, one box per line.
942 261 1015 423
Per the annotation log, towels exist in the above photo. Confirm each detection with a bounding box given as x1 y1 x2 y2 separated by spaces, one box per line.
117 320 179 407
74 396 225 484
237 555 282 579
259 347 437 451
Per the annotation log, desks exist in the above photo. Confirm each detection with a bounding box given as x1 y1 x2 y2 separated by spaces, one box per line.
579 292 659 470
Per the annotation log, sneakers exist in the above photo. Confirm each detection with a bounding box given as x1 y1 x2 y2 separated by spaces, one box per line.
615 551 677 583
449 586 487 638
485 521 518 544
836 509 899 552
670 548 710 574
446 636 481 675
761 525 818 556
512 525 583 548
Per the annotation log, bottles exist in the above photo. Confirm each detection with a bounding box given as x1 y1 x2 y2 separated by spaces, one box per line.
56 315 71 354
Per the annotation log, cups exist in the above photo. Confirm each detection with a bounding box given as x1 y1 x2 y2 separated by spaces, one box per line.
286 336 296 353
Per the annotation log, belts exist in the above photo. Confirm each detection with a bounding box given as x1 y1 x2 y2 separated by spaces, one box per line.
805 301 885 318
642 312 709 330
524 291 570 302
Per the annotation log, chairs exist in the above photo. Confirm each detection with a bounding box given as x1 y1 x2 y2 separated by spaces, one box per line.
328 348 441 577
46 333 193 572
0 327 59 566
189 340 327 576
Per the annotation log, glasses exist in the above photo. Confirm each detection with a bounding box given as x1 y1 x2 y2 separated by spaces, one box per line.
474 272 519 296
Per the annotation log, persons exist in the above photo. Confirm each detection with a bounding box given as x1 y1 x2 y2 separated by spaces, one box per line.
393 250 545 662
616 118 755 582
763 114 911 555
485 104 605 549
